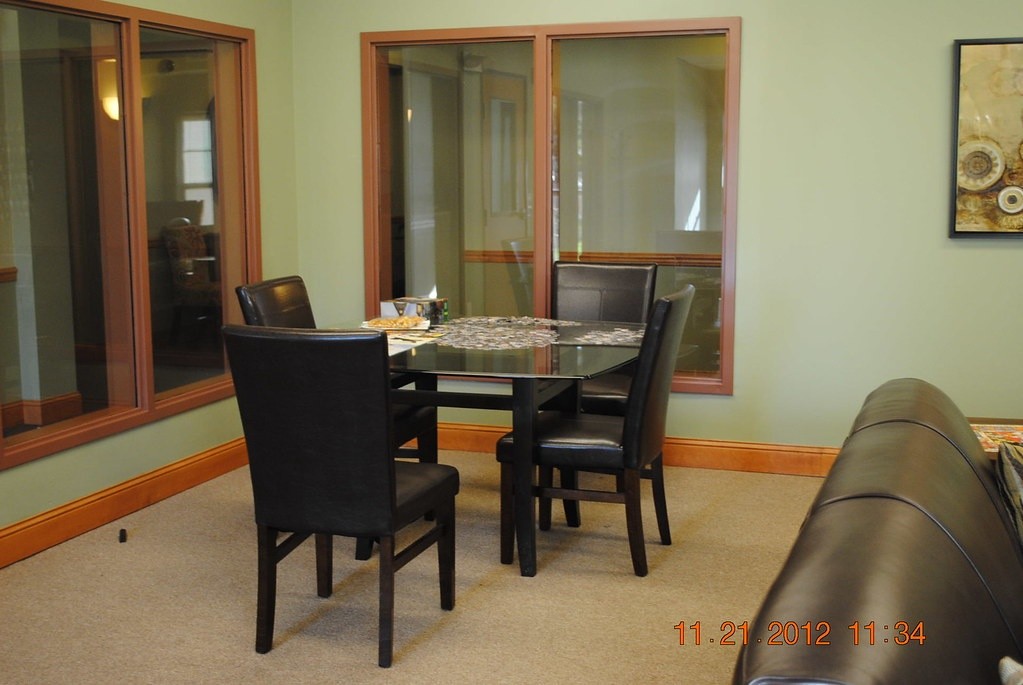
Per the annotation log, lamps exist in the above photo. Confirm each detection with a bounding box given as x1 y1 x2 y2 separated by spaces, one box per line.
96 58 119 122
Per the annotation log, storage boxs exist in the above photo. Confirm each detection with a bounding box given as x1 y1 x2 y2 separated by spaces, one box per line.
380 296 449 326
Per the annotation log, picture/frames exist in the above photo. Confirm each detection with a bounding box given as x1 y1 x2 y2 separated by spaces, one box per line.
950 36 1023 238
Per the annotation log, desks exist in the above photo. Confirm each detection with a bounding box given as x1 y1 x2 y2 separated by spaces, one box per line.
356 314 646 577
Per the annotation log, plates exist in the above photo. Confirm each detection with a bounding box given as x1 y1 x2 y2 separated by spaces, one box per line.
359 319 431 334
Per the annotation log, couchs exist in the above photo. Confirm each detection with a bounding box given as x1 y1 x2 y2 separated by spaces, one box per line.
730 377 1023 684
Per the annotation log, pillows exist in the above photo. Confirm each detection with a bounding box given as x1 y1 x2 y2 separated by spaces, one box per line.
993 442 1023 543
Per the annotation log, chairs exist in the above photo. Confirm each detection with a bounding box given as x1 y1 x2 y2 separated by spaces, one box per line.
495 283 696 577
234 274 436 560
223 323 460 668
164 223 222 348
536 261 658 416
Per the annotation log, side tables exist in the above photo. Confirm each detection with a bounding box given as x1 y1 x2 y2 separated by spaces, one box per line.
965 417 1023 462
